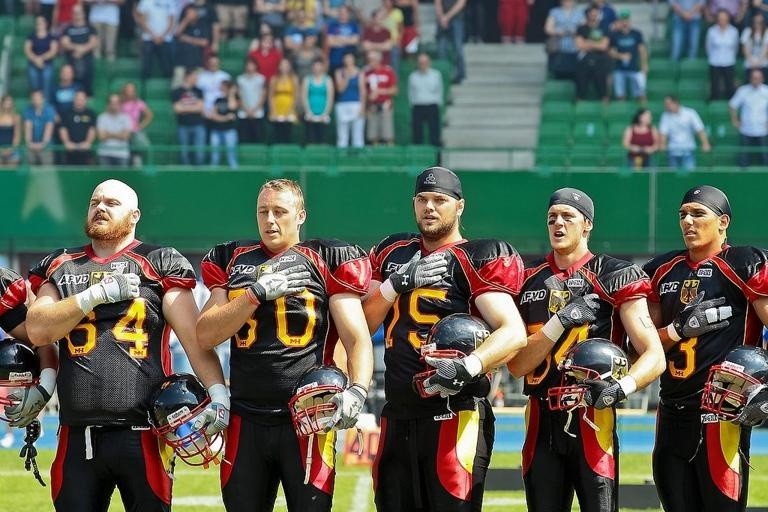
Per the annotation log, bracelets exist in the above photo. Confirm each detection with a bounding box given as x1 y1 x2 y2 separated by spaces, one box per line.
245 289 261 306
207 383 230 412
461 352 483 378
380 279 398 303
351 382 369 395
40 368 56 396
616 374 637 398
541 312 565 342
666 322 683 342
76 283 105 316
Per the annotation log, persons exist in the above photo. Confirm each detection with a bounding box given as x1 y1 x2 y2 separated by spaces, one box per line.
359 168 528 512
196 178 373 512
628 186 768 512
24 180 230 512
544 0 768 169
508 186 667 512
0 267 59 429
0 0 529 172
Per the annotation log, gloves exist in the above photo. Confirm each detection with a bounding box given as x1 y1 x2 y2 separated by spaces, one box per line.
323 387 367 435
585 380 624 409
248 259 312 304
77 269 143 319
733 385 762 428
379 245 450 304
666 290 732 342
542 293 601 340
2 369 59 429
421 356 483 400
192 384 231 436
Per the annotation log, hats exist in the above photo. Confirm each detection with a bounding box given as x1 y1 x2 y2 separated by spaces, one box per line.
681 184 732 225
414 166 464 202
547 338 629 412
548 186 596 222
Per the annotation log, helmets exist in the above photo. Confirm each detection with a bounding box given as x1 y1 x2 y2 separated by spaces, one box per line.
701 342 764 425
0 338 38 426
152 373 226 467
286 363 347 439
410 313 495 401
